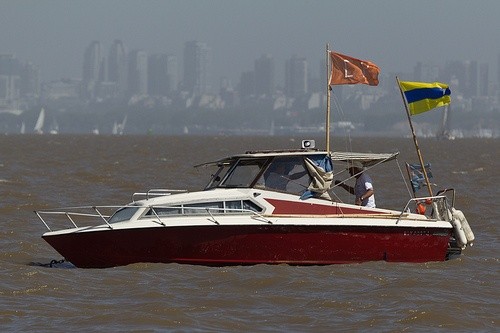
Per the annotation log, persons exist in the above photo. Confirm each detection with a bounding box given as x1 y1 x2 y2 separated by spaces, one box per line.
334 161 377 208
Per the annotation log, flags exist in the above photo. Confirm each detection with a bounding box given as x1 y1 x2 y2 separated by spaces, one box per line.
329 50 380 87
406 162 437 193
399 81 451 117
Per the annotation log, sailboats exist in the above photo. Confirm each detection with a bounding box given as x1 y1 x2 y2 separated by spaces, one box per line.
112 120 121 136
266 120 277 135
183 126 192 134
32 110 47 136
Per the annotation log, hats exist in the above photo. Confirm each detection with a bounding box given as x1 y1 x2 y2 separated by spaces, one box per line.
345 163 358 168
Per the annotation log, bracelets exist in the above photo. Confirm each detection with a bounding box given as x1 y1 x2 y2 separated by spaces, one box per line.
360 196 364 201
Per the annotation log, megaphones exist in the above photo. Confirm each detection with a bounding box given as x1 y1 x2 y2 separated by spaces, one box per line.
301 140 316 148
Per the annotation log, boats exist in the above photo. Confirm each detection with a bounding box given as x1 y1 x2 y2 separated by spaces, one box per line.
31 43 474 264
